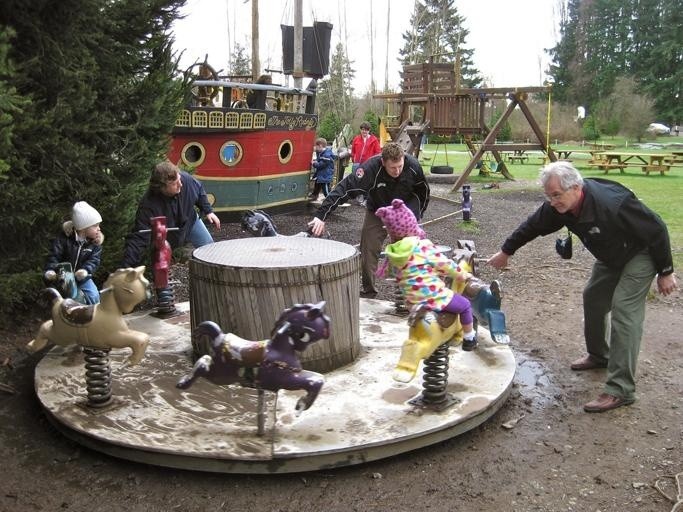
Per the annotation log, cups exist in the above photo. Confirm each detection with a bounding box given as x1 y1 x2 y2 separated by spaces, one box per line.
542 187 570 202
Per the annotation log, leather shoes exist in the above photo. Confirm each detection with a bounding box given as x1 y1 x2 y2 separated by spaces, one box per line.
44 270 56 281
74 268 87 281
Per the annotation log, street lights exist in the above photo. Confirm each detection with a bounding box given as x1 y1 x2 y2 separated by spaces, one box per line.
556 230 573 259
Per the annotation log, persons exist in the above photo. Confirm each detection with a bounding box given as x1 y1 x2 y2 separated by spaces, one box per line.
308 140 430 312
462 186 473 209
44 200 104 305
308 138 338 198
110 161 222 268
350 122 383 205
484 160 677 412
374 199 479 351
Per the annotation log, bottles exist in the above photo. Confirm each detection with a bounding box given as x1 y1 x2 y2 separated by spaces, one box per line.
374 199 427 242
71 201 102 231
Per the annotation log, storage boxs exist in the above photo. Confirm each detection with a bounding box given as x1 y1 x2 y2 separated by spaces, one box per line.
475 130 683 175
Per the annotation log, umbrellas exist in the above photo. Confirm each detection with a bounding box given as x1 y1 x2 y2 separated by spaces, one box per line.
583 393 635 412
570 353 607 370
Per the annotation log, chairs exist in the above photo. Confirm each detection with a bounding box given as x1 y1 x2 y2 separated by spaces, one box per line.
461 337 480 351
358 199 368 207
308 193 317 201
355 194 364 203
